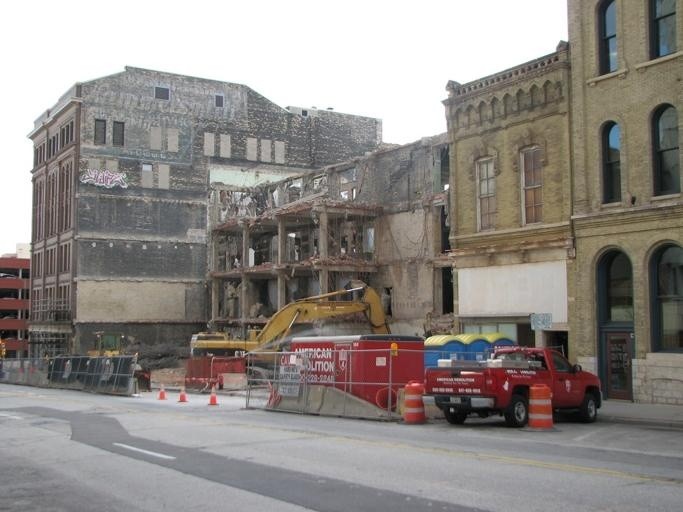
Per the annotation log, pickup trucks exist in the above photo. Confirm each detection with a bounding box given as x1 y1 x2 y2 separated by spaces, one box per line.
419 345 603 427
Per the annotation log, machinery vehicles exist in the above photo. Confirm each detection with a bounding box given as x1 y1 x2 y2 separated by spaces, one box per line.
183 278 393 391
86 330 152 393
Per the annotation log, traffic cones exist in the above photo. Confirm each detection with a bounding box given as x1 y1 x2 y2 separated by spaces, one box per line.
177 385 187 402
207 384 219 405
156 383 166 400
